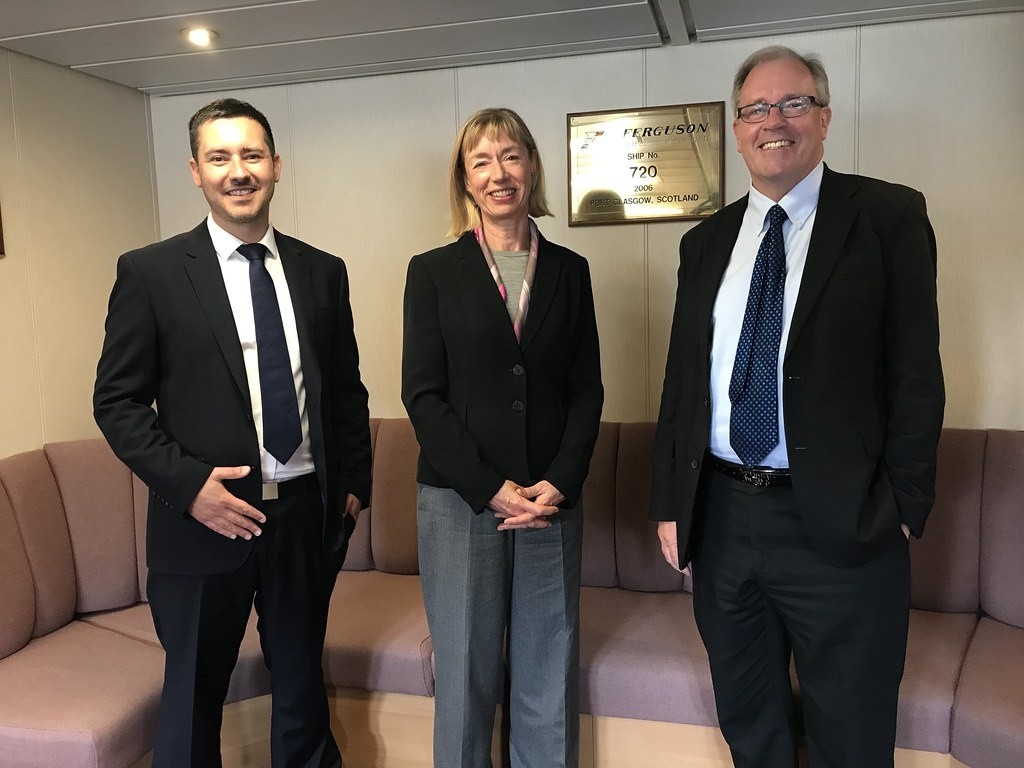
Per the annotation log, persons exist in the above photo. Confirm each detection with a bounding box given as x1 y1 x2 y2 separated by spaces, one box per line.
646 46 946 768
401 107 608 768
92 98 373 768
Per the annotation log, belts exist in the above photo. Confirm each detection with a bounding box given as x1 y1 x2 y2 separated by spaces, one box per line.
262 475 319 500
705 451 792 489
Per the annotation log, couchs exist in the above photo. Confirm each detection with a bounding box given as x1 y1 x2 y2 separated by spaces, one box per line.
0 421 1024 768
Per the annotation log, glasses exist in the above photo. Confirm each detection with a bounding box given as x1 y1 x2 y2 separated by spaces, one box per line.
736 95 822 123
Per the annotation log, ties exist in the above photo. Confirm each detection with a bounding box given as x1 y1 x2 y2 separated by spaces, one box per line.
729 204 789 471
235 242 302 465
